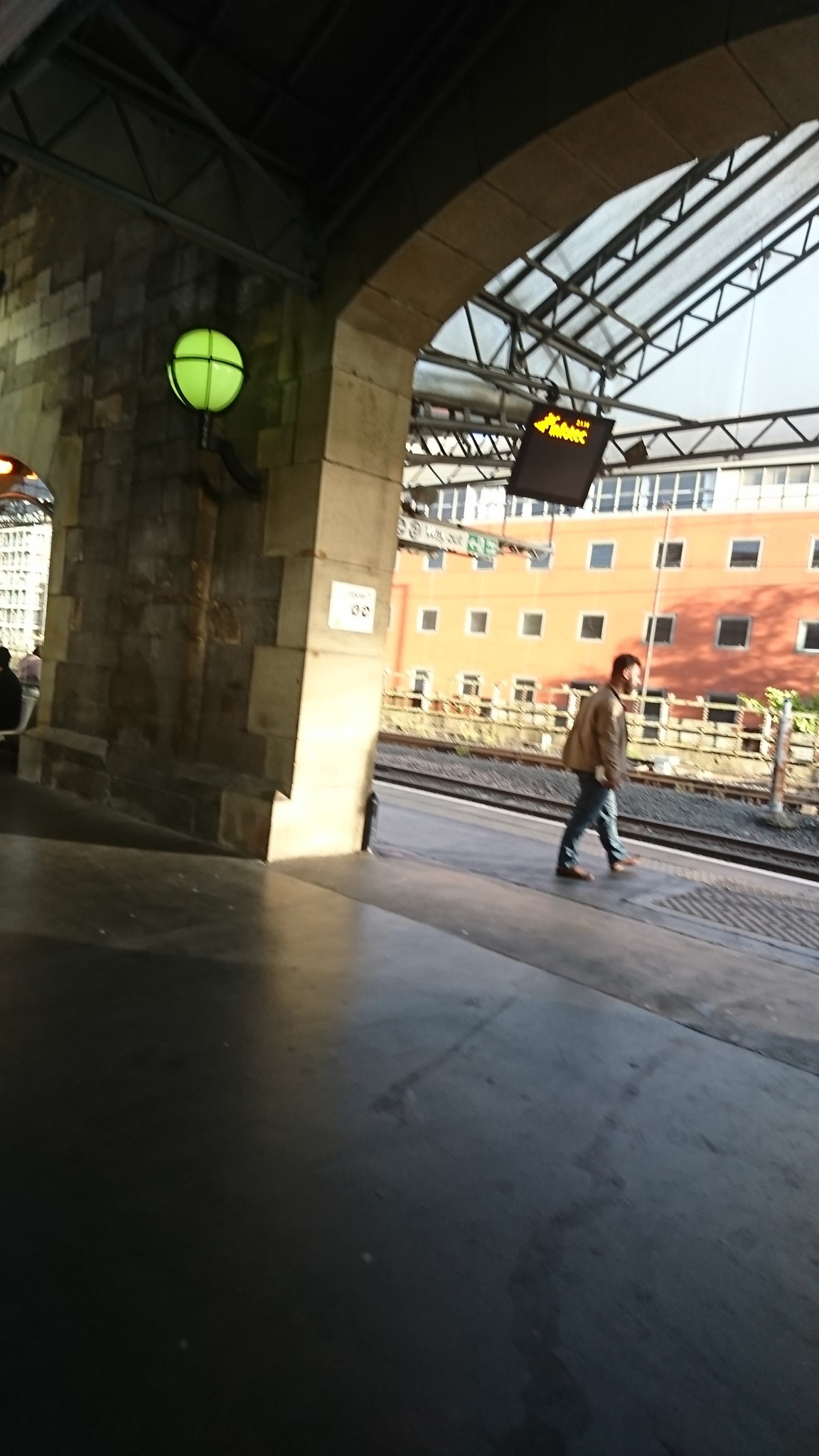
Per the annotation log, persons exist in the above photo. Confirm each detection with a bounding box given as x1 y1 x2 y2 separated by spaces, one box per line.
555 654 644 881
0 647 23 776
16 646 42 685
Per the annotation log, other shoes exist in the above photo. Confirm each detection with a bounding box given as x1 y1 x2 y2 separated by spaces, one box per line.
557 866 593 881
611 853 641 873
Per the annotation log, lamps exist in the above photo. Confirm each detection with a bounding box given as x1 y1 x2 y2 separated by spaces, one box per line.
164 324 266 497
